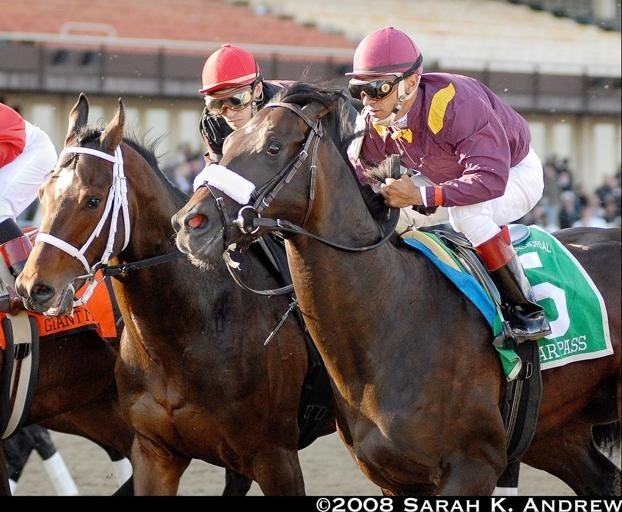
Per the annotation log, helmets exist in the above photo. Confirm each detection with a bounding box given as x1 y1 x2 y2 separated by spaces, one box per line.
344 27 423 76
199 44 260 93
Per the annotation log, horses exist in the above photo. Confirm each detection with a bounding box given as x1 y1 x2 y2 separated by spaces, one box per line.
0 65 622 496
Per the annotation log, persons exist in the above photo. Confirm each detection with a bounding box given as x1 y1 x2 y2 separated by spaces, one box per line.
158 136 205 196
343 25 552 341
508 151 621 227
0 104 75 317
197 43 293 162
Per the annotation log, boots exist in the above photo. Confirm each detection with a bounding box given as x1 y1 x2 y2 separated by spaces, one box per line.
472 224 554 350
0 234 39 315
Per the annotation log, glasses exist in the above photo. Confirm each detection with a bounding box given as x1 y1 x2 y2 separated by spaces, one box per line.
348 56 422 102
204 74 265 115
